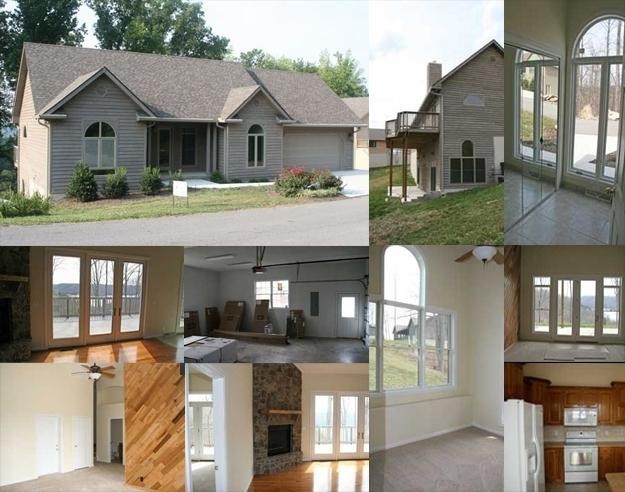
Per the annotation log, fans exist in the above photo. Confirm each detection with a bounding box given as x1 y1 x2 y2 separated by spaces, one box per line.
454 244 505 268
72 363 117 383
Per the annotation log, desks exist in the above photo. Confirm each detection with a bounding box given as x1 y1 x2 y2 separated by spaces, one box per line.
184 335 238 363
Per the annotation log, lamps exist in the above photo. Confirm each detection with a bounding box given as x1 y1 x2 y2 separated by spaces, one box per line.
471 245 497 266
89 373 102 382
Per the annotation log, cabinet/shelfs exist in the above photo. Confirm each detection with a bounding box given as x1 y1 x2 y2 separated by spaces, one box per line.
528 379 547 405
599 389 625 426
504 362 525 399
566 387 598 408
543 447 564 486
544 390 565 427
598 447 625 479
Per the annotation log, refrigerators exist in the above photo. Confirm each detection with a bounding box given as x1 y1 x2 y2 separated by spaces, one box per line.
504 398 545 492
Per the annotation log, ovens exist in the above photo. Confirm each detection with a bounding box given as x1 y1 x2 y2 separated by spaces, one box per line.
564 442 598 483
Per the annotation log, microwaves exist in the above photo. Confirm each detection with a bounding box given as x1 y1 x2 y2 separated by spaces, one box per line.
564 408 598 426
